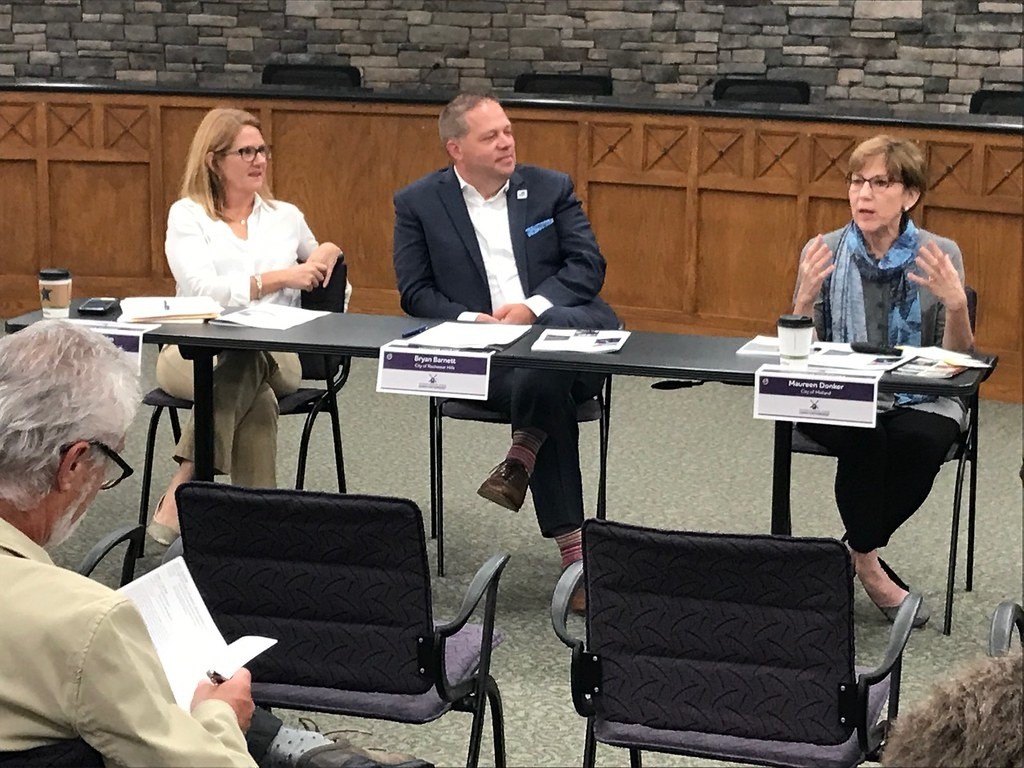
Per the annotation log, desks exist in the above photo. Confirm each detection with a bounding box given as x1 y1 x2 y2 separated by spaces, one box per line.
0 77 1023 405
4 296 999 629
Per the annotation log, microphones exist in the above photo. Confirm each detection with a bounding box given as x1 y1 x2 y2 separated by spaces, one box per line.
690 78 717 99
192 58 200 87
416 63 441 93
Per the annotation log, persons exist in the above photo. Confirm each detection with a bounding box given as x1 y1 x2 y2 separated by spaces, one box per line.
145 108 352 547
391 89 619 614
791 135 975 628
1 312 437 768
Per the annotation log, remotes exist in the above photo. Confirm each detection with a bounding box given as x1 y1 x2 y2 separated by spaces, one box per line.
850 341 903 358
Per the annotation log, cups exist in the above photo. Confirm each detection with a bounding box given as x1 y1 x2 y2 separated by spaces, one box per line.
775 314 816 360
37 268 73 319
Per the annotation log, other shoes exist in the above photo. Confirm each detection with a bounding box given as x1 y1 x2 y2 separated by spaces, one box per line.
146 491 181 547
858 555 930 628
839 531 858 577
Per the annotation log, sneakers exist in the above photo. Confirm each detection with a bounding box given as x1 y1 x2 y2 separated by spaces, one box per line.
570 576 587 616
478 459 531 513
294 716 436 768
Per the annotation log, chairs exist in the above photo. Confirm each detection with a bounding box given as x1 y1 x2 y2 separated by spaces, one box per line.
713 78 811 106
968 90 1024 117
174 481 512 768
551 518 924 767
515 73 613 95
262 64 362 87
425 318 624 575
772 288 980 633
138 259 348 556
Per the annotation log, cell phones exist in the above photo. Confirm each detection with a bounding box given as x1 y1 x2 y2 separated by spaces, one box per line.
78 297 117 315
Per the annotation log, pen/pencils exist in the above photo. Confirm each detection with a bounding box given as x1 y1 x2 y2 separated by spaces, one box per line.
207 669 228 686
402 325 428 338
810 348 821 351
164 300 170 309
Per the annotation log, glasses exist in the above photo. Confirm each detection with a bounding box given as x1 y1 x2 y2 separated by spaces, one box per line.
58 438 134 489
844 171 906 193
212 144 274 162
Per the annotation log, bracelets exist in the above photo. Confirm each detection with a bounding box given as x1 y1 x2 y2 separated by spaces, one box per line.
253 272 263 301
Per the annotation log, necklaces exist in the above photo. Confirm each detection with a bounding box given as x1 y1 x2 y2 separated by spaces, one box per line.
224 212 251 226
